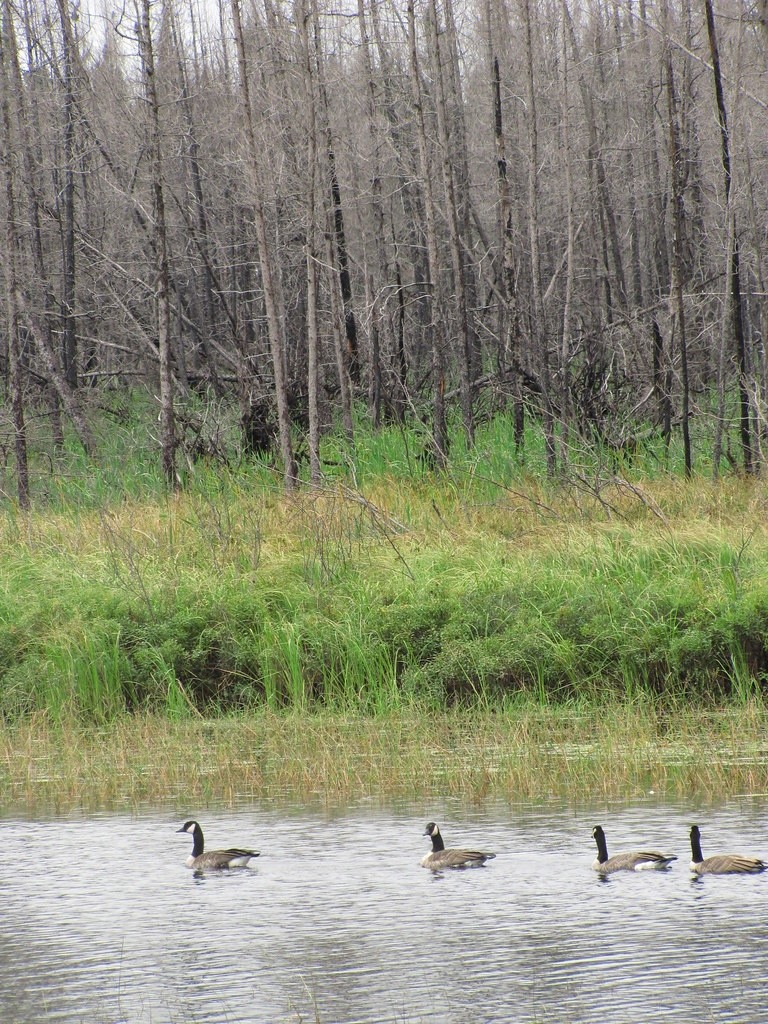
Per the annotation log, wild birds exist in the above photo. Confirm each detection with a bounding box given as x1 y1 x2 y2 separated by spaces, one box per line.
419 822 496 870
686 825 768 874
591 825 679 874
175 821 261 870
